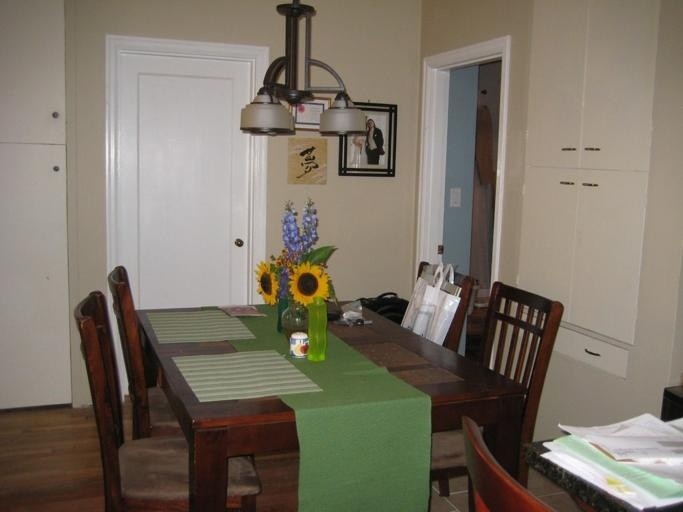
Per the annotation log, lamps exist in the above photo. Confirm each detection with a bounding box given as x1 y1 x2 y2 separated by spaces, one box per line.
240 0 367 135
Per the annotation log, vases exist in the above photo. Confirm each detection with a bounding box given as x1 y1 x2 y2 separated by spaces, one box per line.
280 300 309 343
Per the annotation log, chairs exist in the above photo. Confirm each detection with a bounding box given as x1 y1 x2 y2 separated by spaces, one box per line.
74 290 262 512
462 415 556 511
107 266 185 440
417 262 474 353
430 280 564 512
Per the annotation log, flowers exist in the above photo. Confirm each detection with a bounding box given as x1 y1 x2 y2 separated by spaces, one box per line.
255 197 338 306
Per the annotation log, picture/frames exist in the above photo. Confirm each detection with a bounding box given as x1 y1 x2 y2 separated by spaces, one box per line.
289 97 332 131
339 102 397 176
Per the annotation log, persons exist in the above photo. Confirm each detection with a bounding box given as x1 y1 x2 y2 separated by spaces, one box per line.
365 117 384 166
352 134 368 166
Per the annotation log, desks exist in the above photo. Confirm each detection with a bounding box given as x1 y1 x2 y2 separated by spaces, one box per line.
525 417 683 511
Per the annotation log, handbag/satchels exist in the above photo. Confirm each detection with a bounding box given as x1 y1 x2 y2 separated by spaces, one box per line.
400 277 461 346
361 293 408 325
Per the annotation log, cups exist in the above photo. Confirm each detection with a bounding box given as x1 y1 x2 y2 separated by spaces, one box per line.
289 332 308 358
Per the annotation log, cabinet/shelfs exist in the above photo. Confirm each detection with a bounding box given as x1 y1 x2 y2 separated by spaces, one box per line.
0 0 74 410
515 0 661 381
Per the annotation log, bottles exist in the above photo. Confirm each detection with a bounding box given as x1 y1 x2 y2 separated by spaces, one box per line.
307 296 328 362
